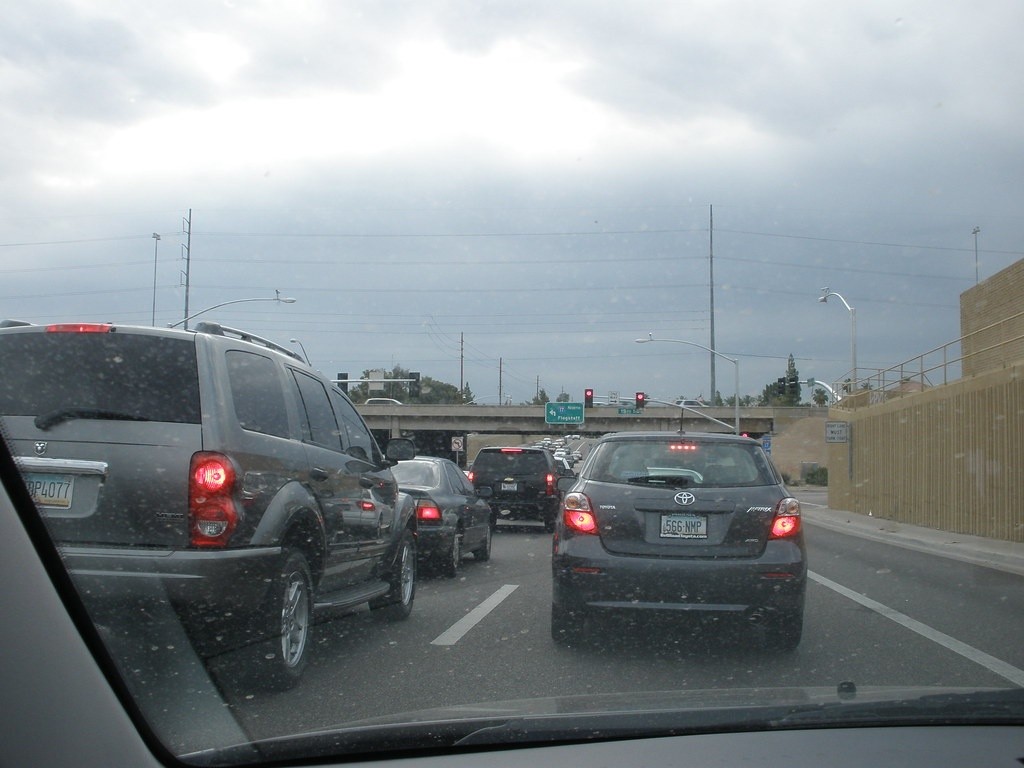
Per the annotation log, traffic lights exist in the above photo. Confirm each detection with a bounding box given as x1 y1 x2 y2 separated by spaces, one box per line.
740 432 749 437
585 389 593 410
636 392 645 408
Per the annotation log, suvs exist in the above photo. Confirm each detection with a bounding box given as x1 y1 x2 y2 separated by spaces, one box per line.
0 317 421 692
549 430 809 649
466 445 574 534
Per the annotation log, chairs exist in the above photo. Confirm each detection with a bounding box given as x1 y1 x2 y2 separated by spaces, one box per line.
613 456 649 485
704 466 732 484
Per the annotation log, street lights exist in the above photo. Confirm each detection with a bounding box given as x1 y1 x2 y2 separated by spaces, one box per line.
972 222 981 285
165 296 299 328
290 339 313 366
152 230 164 322
633 332 741 435
818 291 857 393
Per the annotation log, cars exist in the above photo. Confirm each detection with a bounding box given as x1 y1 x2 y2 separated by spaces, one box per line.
379 454 497 579
674 400 710 408
461 470 471 476
363 397 403 406
517 433 583 470
